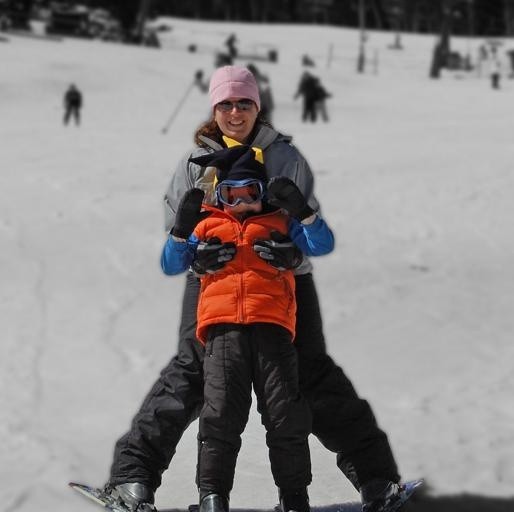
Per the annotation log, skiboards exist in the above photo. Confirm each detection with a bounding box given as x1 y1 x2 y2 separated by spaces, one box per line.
69 479 424 512
67 480 158 512
359 479 424 512
188 492 230 512
272 486 311 512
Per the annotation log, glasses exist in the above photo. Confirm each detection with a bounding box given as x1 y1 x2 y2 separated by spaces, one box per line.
215 177 264 204
217 99 251 110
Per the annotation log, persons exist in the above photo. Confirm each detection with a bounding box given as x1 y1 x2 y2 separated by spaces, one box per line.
227 34 238 58
248 65 273 113
489 47 501 89
62 83 82 124
430 34 450 79
294 73 329 123
107 65 406 512
160 135 334 512
196 54 232 95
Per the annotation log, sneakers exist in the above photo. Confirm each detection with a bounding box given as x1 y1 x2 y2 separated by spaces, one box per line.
116 481 154 511
360 476 398 510
200 495 227 511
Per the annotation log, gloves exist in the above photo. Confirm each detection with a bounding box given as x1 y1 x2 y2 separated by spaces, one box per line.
266 177 314 222
169 190 213 241
252 230 303 269
191 236 235 276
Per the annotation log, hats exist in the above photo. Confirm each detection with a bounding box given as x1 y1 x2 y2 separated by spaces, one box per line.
187 145 266 188
210 65 262 111
